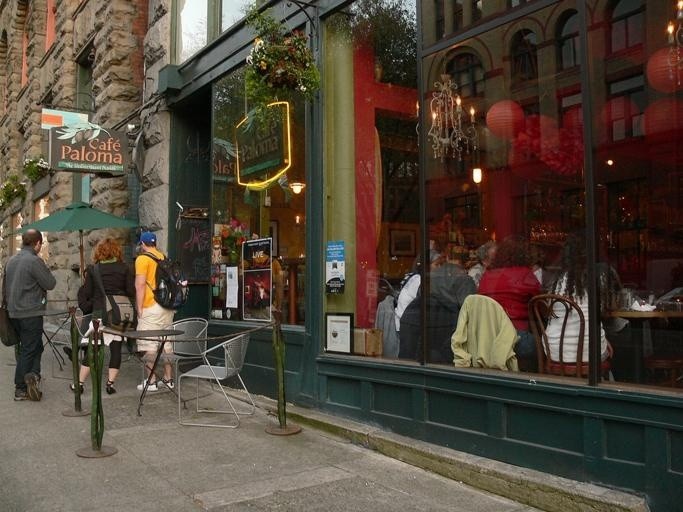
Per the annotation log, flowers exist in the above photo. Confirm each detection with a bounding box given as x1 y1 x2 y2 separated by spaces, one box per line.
1 166 23 206
234 4 322 136
218 216 258 251
21 154 50 183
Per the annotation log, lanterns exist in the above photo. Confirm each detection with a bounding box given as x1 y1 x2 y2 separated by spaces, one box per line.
486 45 683 184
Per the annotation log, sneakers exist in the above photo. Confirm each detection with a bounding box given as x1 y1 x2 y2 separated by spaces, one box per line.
23 369 44 402
13 387 29 402
136 378 159 392
157 377 175 390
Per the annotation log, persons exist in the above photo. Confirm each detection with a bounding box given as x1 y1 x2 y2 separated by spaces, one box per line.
2 228 57 399
237 241 283 323
395 230 683 382
63 232 176 394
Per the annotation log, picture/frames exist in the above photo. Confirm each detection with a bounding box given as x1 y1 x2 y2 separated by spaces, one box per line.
268 219 279 256
240 237 271 322
389 229 416 257
323 311 354 356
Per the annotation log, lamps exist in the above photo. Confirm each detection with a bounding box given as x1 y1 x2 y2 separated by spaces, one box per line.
414 74 478 163
126 116 150 135
286 170 306 195
483 41 682 182
663 1 683 87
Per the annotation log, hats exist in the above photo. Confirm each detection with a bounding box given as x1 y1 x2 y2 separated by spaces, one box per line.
135 231 157 247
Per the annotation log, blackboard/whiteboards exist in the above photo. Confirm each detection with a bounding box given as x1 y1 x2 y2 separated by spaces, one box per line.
177 216 213 285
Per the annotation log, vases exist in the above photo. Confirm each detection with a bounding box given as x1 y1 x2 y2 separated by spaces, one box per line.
257 64 302 89
227 244 239 267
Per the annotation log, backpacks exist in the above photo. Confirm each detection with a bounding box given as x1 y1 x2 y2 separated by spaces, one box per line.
246 256 276 308
138 251 191 310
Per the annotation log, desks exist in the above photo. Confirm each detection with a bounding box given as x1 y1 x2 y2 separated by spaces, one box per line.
274 256 305 325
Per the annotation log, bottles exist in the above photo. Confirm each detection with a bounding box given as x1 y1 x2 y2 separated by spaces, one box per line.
632 290 680 313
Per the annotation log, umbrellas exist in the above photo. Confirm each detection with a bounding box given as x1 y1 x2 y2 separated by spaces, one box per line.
4 200 161 288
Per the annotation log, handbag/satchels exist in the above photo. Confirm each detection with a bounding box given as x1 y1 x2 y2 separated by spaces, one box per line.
103 291 139 327
0 302 21 348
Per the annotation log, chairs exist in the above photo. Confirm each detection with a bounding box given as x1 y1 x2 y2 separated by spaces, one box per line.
400 296 457 363
453 295 519 371
527 294 611 382
373 296 403 358
33 303 258 436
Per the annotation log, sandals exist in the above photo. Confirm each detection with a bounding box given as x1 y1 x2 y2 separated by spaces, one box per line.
69 380 85 394
105 378 117 395
